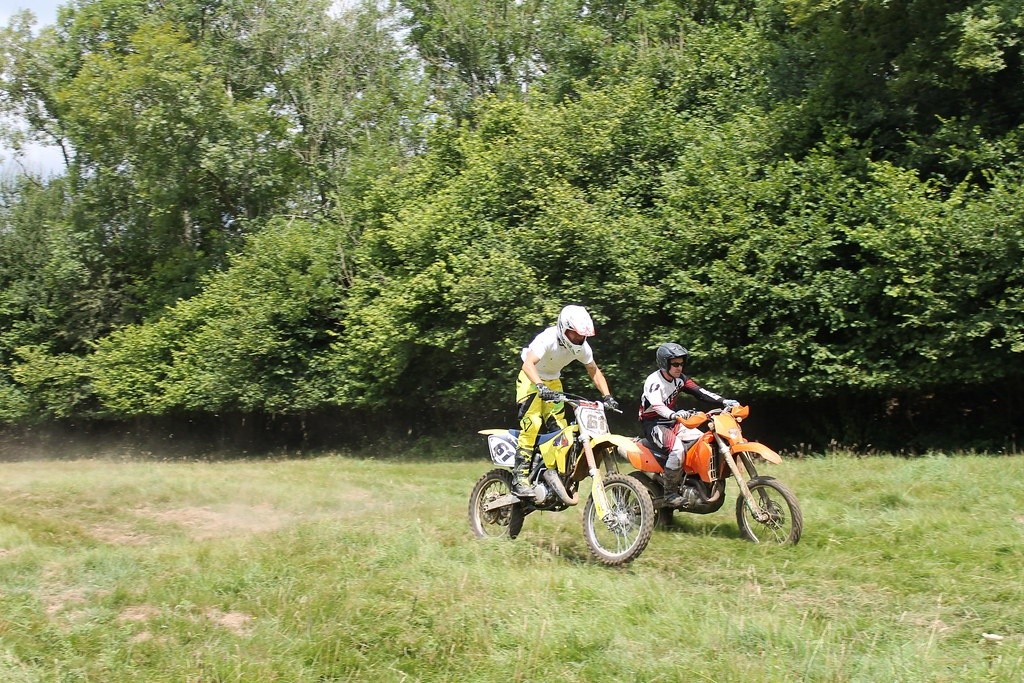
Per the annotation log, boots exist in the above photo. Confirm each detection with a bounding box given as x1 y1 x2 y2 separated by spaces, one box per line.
510 447 537 498
662 466 689 507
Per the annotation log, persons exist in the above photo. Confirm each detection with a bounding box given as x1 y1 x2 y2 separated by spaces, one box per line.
510 305 618 498
639 343 740 508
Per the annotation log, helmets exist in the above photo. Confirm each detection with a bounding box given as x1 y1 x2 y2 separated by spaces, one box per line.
555 304 595 355
655 342 688 370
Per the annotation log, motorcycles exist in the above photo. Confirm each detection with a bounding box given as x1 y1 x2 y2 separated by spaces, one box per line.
468 389 654 566
621 401 803 547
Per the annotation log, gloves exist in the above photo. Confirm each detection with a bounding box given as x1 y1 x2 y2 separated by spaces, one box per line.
536 384 554 400
724 399 740 411
673 409 691 420
603 395 619 412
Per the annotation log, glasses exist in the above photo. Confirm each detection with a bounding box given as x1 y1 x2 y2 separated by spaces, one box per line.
670 361 685 367
